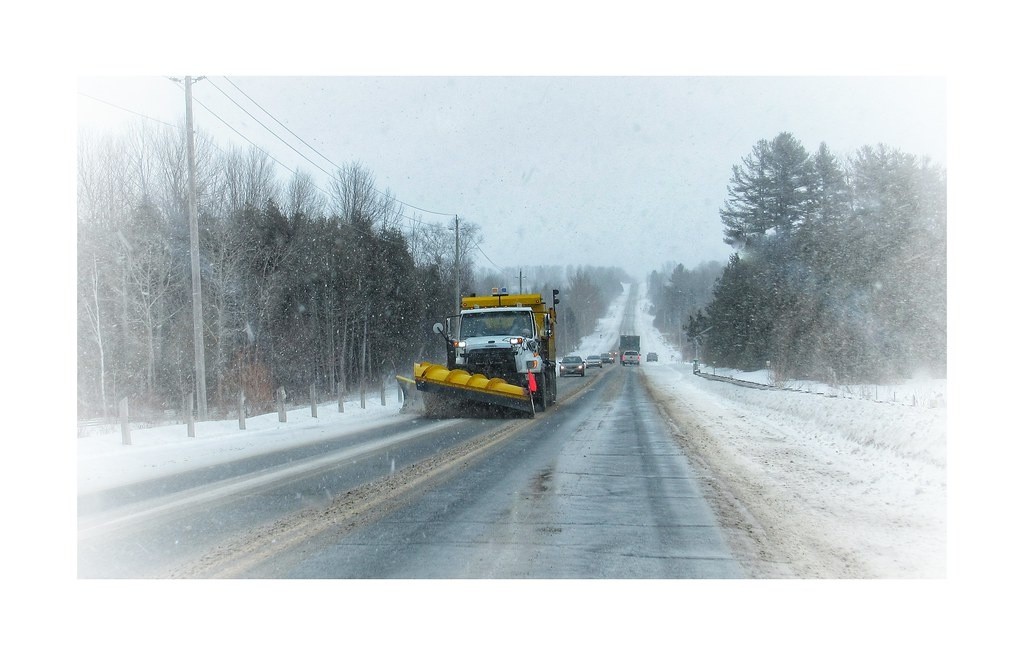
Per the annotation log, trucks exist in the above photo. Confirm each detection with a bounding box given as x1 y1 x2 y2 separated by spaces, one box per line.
619 334 641 364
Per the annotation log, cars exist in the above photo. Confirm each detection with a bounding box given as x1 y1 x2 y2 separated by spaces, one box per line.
585 354 603 368
601 349 618 364
619 350 642 366
558 355 586 377
646 352 659 363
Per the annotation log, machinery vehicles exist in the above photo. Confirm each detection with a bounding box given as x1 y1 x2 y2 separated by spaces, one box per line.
397 286 561 419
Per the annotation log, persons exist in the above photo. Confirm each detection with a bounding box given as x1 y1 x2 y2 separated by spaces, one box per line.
513 314 525 327
